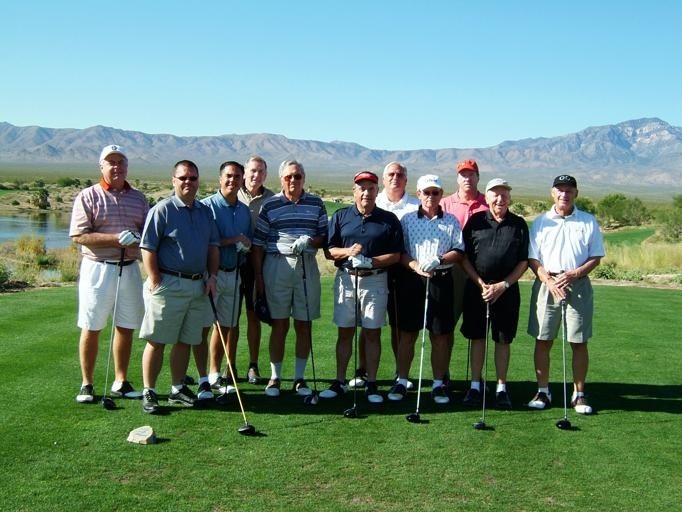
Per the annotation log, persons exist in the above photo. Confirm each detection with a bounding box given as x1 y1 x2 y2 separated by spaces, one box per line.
69 145 150 403
528 175 605 415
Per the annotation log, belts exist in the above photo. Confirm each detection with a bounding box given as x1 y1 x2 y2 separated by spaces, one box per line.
157 265 204 280
344 268 386 276
550 272 589 279
94 258 135 266
218 266 236 272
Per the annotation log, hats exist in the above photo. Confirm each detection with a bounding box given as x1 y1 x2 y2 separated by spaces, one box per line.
354 170 378 183
416 173 443 191
486 178 511 191
456 160 479 174
99 145 128 160
552 175 577 188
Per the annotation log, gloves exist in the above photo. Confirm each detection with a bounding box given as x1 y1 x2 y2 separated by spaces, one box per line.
418 256 440 272
290 234 310 255
119 229 141 247
348 255 373 268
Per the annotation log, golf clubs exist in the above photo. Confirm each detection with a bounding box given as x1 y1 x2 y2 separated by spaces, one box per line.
215 249 242 406
556 300 571 430
343 268 362 418
101 248 125 409
406 278 430 423
204 280 255 433
473 301 489 430
300 250 319 404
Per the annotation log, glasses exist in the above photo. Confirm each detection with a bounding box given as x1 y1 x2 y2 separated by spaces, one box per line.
282 174 304 180
419 190 441 196
174 175 200 181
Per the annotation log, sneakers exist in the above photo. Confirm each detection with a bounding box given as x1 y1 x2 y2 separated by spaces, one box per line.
74 372 596 415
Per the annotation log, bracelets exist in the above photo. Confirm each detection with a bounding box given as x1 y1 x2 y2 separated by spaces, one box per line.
544 276 552 286
